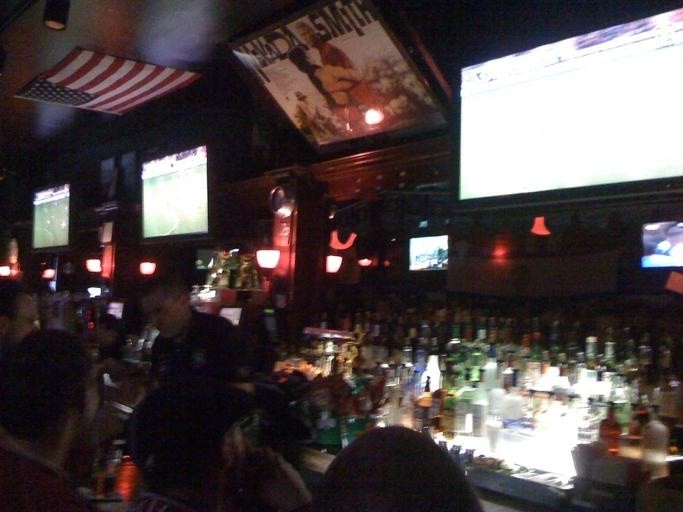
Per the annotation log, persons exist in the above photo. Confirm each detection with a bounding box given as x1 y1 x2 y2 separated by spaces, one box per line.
294 20 355 69
287 46 370 107
311 426 484 512
0 279 42 355
139 275 256 395
124 380 311 512
0 327 105 512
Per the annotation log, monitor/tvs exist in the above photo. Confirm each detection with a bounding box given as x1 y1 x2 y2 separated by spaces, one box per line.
458 8 683 210
32 182 70 249
140 144 209 238
641 220 683 268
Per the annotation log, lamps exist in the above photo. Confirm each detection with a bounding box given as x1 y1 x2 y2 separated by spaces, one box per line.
256 250 280 281
43 0 70 31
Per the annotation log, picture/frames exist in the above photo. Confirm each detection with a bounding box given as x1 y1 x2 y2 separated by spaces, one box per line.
222 0 451 163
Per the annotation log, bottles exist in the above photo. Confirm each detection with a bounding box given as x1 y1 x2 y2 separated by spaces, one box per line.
125 322 161 361
91 454 137 512
271 303 673 481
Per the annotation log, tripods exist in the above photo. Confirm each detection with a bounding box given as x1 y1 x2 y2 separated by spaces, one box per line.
408 235 449 271
